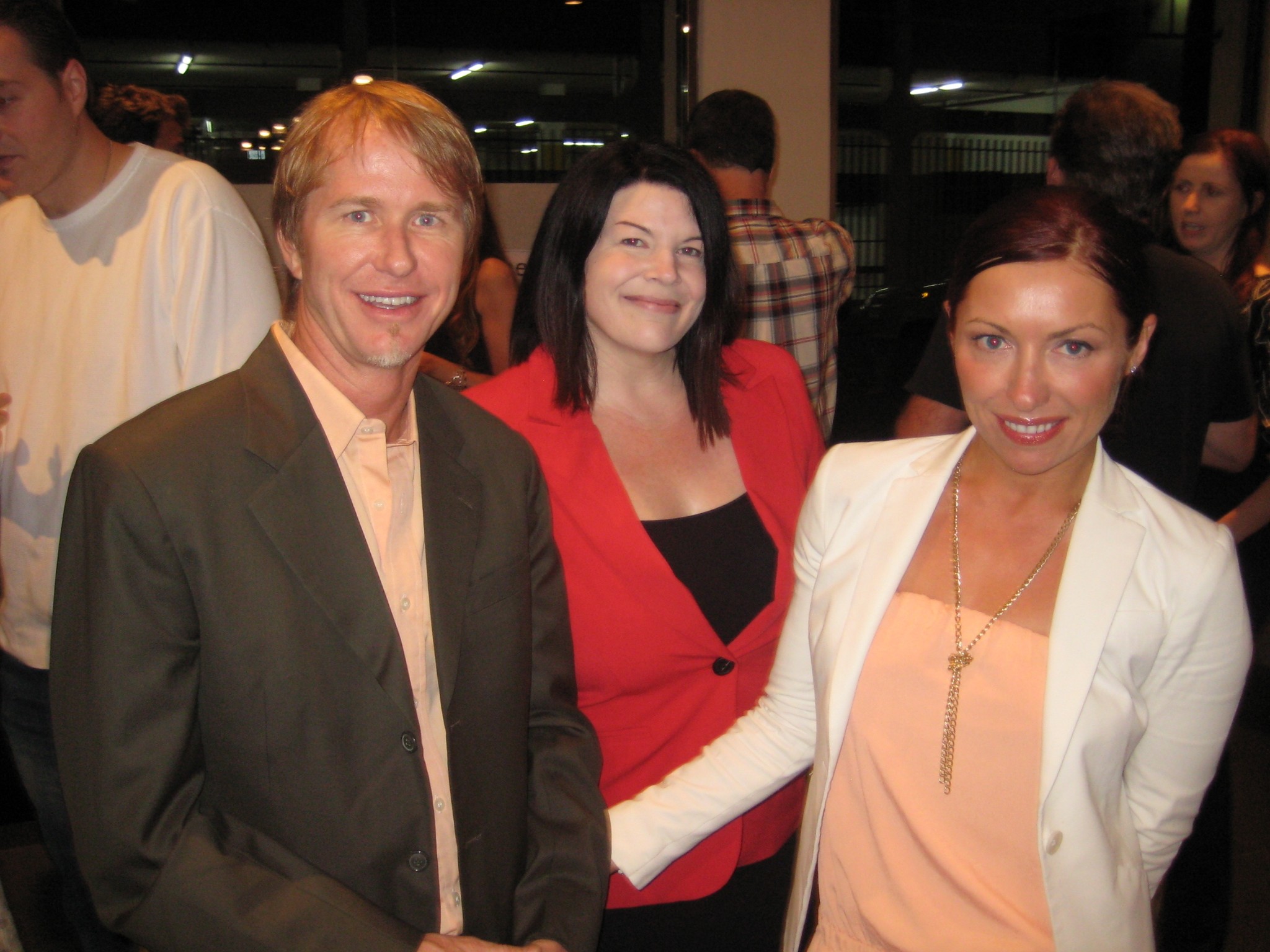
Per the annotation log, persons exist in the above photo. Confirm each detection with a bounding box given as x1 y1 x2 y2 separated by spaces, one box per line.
604 190 1255 952
0 0 284 952
688 89 854 439
890 72 1270 952
1168 130 1270 554
462 143 823 952
93 83 189 158
424 199 519 391
51 79 608 952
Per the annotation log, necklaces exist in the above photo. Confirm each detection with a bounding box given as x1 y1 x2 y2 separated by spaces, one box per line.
938 444 1083 794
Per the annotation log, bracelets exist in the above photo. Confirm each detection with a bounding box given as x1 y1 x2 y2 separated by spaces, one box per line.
445 365 467 390
